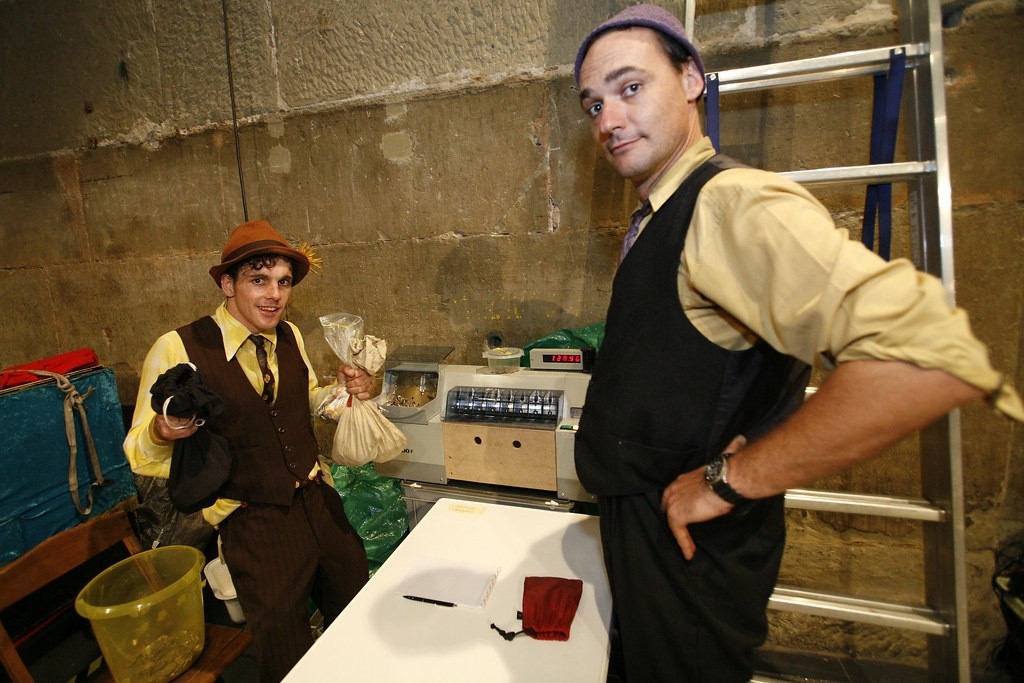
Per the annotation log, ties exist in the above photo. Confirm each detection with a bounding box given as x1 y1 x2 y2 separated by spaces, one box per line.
248 334 275 404
619 203 652 265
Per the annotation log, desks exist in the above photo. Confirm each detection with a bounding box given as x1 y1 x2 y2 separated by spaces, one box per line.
279 497 612 682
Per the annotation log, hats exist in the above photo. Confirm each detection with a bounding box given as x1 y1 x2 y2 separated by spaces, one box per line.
574 4 706 103
209 221 311 290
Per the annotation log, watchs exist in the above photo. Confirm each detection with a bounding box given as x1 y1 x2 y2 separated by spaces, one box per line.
704 452 752 510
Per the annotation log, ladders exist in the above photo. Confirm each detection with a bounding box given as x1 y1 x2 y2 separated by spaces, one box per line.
670 0 982 683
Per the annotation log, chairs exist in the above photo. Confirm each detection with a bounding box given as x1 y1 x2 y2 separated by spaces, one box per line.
0 508 252 682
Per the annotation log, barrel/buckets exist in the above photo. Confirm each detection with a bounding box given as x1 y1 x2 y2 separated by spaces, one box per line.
75 545 206 683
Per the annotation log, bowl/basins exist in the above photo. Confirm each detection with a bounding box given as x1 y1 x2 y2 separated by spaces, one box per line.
482 348 525 374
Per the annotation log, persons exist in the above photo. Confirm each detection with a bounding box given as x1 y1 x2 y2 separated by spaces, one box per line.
123 219 375 683
574 3 1002 683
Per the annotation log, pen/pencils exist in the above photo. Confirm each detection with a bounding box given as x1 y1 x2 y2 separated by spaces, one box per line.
402 595 457 608
